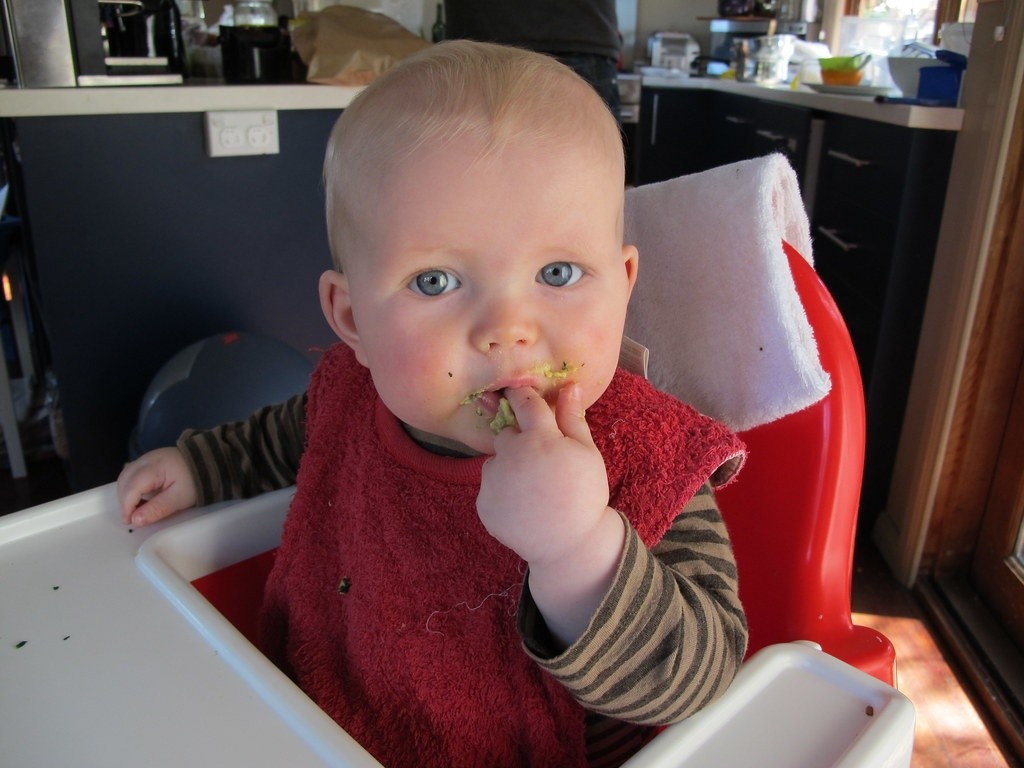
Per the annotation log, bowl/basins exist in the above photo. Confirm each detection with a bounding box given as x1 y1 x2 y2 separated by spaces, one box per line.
888 56 948 98
735 33 794 86
816 56 863 86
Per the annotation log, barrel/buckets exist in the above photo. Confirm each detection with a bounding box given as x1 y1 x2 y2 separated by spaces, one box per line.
937 22 977 58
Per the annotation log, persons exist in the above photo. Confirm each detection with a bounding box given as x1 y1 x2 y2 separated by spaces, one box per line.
442 0 624 133
112 38 748 767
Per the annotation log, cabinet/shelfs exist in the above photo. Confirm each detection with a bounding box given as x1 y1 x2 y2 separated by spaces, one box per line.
636 84 815 214
811 114 958 546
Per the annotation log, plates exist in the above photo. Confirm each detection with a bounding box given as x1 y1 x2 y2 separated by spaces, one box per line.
806 82 892 98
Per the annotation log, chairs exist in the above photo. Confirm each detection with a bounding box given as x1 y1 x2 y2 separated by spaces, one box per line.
1 239 911 766
